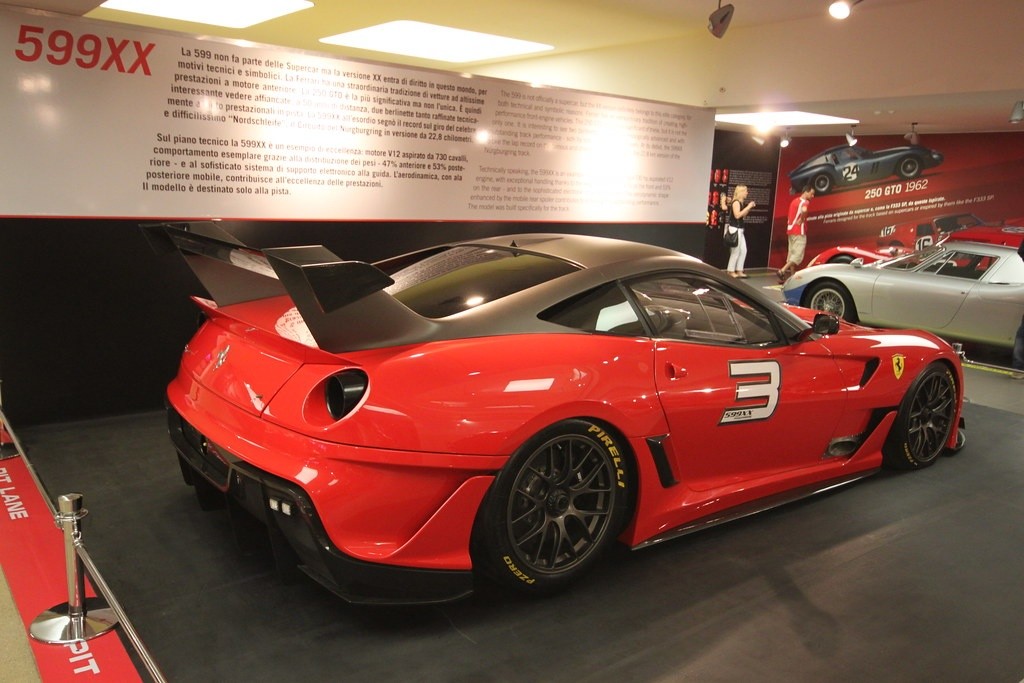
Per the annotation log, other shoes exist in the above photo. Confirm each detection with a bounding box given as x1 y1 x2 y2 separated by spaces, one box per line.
737 272 747 277
727 272 738 277
776 270 784 284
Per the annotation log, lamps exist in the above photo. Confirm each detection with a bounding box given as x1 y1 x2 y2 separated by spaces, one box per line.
780 127 791 148
846 124 858 146
706 0 736 37
829 0 861 18
1008 101 1024 124
751 135 766 146
903 123 920 144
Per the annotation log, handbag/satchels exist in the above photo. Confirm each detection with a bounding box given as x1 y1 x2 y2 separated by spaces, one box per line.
725 227 738 247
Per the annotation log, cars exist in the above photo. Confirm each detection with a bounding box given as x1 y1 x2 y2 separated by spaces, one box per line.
874 210 1023 255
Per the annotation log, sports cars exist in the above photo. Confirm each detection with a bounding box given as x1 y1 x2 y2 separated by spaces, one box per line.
139 220 964 608
781 226 1024 369
788 145 943 196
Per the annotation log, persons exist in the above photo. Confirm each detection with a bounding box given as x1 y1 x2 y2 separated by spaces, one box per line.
775 185 814 284
724 185 756 278
